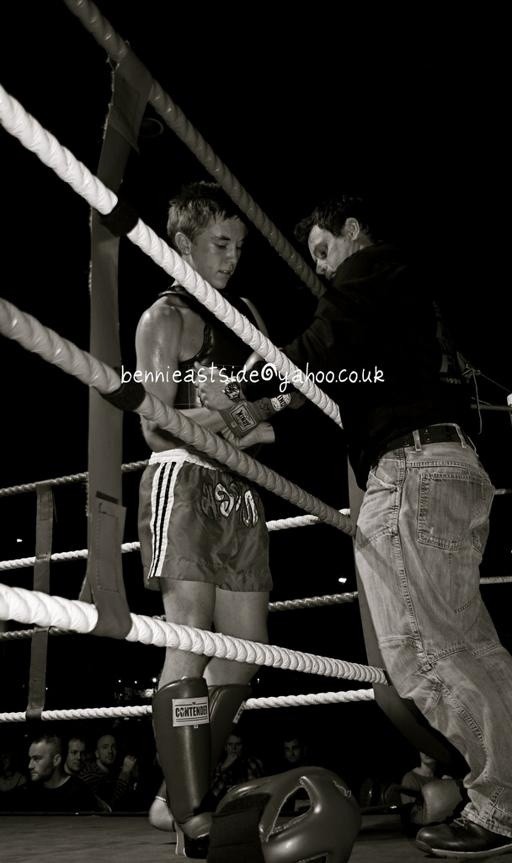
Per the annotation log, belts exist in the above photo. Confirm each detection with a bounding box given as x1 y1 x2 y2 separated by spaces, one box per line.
372 425 475 470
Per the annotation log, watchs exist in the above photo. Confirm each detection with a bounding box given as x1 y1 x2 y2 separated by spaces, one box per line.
225 379 245 404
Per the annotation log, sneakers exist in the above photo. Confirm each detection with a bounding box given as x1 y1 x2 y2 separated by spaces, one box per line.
148 794 177 832
415 814 512 862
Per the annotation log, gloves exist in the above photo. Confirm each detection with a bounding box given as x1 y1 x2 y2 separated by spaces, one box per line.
238 343 307 404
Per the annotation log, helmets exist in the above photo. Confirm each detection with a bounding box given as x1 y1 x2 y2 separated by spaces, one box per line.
205 765 364 862
410 774 465 826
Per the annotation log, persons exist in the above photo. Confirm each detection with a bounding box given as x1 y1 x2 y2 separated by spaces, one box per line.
209 734 274 808
0 726 166 819
133 182 274 832
280 733 320 769
194 195 512 862
